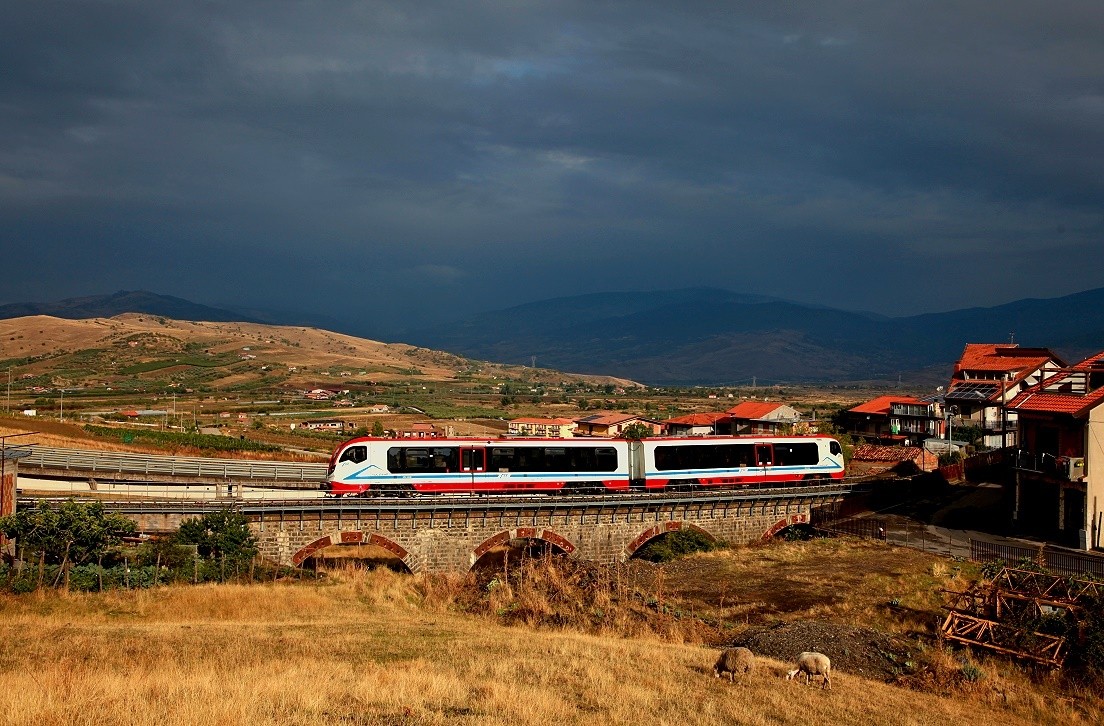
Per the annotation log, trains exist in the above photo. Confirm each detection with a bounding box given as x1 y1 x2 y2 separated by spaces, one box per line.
318 425 846 495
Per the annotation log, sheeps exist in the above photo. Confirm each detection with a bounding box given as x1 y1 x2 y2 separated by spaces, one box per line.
713 647 754 685
786 652 831 691
